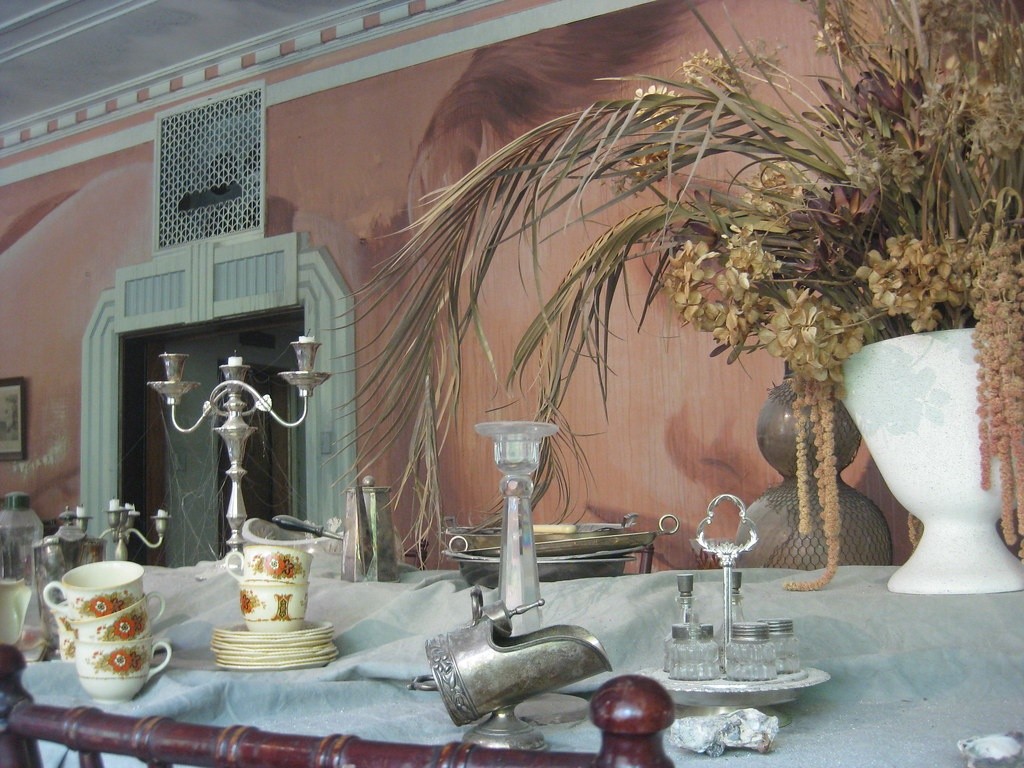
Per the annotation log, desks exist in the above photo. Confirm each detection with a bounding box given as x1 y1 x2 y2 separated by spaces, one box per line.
15 560 1024 767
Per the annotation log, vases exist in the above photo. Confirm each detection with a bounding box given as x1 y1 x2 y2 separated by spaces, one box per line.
835 328 1024 595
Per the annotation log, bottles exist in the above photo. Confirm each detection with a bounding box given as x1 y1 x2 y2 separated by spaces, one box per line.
663 572 800 680
0 491 46 661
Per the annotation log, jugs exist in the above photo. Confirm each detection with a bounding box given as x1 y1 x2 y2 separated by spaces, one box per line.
340 475 400 583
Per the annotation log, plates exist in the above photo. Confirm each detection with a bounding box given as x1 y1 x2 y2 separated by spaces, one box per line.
209 619 339 672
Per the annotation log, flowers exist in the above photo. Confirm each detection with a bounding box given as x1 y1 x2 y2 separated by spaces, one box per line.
307 0 1024 591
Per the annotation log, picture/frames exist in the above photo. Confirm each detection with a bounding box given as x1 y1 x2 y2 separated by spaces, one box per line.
0 376 24 461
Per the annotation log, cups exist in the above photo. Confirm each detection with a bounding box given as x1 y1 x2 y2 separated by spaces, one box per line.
42 560 172 706
226 545 314 632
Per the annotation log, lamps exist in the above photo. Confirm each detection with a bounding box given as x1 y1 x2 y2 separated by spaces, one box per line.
148 334 334 579
